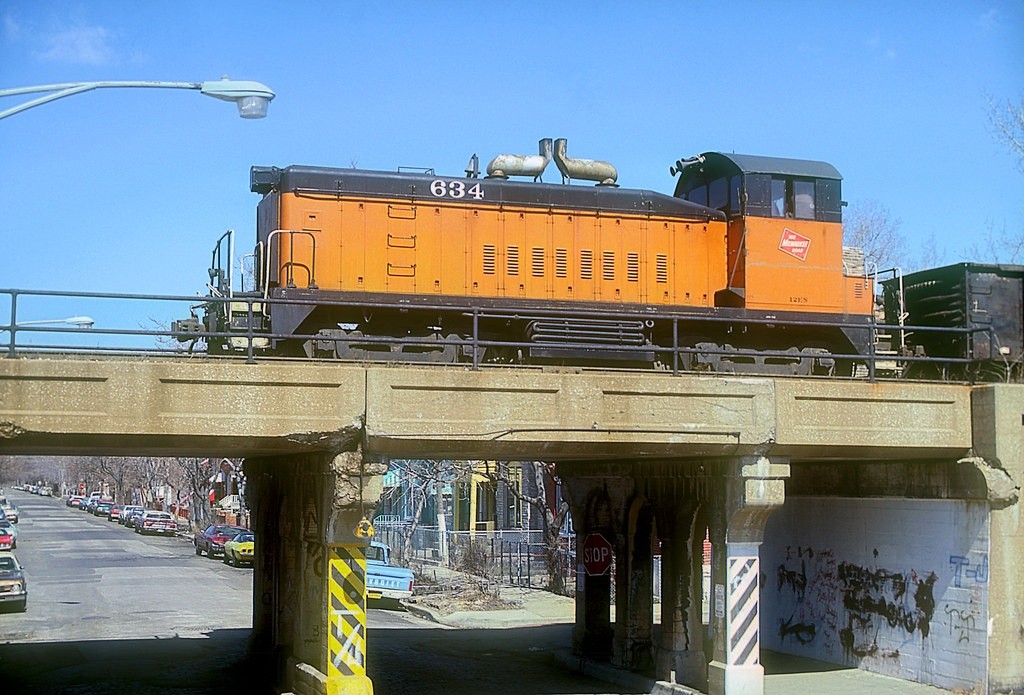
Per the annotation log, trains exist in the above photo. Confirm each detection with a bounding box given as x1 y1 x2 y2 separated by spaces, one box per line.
167 136 1022 383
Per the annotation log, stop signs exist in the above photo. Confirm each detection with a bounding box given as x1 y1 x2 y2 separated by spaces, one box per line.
581 532 614 577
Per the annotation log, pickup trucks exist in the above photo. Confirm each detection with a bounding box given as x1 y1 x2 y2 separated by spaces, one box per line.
365 541 415 602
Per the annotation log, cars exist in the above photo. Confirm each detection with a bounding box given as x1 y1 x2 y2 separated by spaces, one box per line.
66 490 144 528
223 532 255 567
193 524 248 559
0 551 29 611
136 511 178 537
0 485 22 550
9 484 53 497
